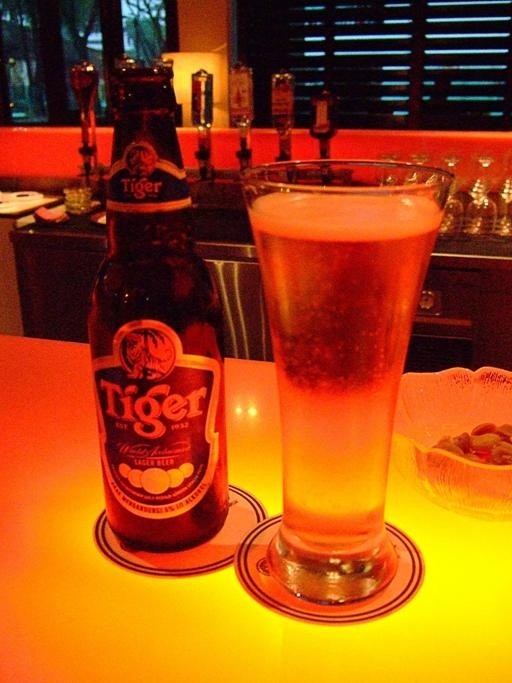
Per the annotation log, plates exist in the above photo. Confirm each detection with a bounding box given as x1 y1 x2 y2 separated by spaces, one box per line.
8 200 512 373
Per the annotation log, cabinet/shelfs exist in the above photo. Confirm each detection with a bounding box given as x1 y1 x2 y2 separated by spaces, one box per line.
271 71 294 161
189 68 214 160
86 65 230 553
308 88 336 160
227 65 255 152
70 59 102 155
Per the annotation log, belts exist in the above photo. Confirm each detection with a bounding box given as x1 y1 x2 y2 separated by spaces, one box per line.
380 151 512 242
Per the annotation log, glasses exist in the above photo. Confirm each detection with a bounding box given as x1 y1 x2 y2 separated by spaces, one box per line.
160 52 230 105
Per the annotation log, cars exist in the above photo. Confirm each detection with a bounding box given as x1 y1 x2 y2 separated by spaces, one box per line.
63 186 92 215
236 155 456 610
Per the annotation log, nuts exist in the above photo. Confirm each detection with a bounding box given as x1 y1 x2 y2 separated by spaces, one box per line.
434 423 512 465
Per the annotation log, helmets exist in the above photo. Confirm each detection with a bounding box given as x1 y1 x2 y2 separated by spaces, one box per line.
392 363 512 511
